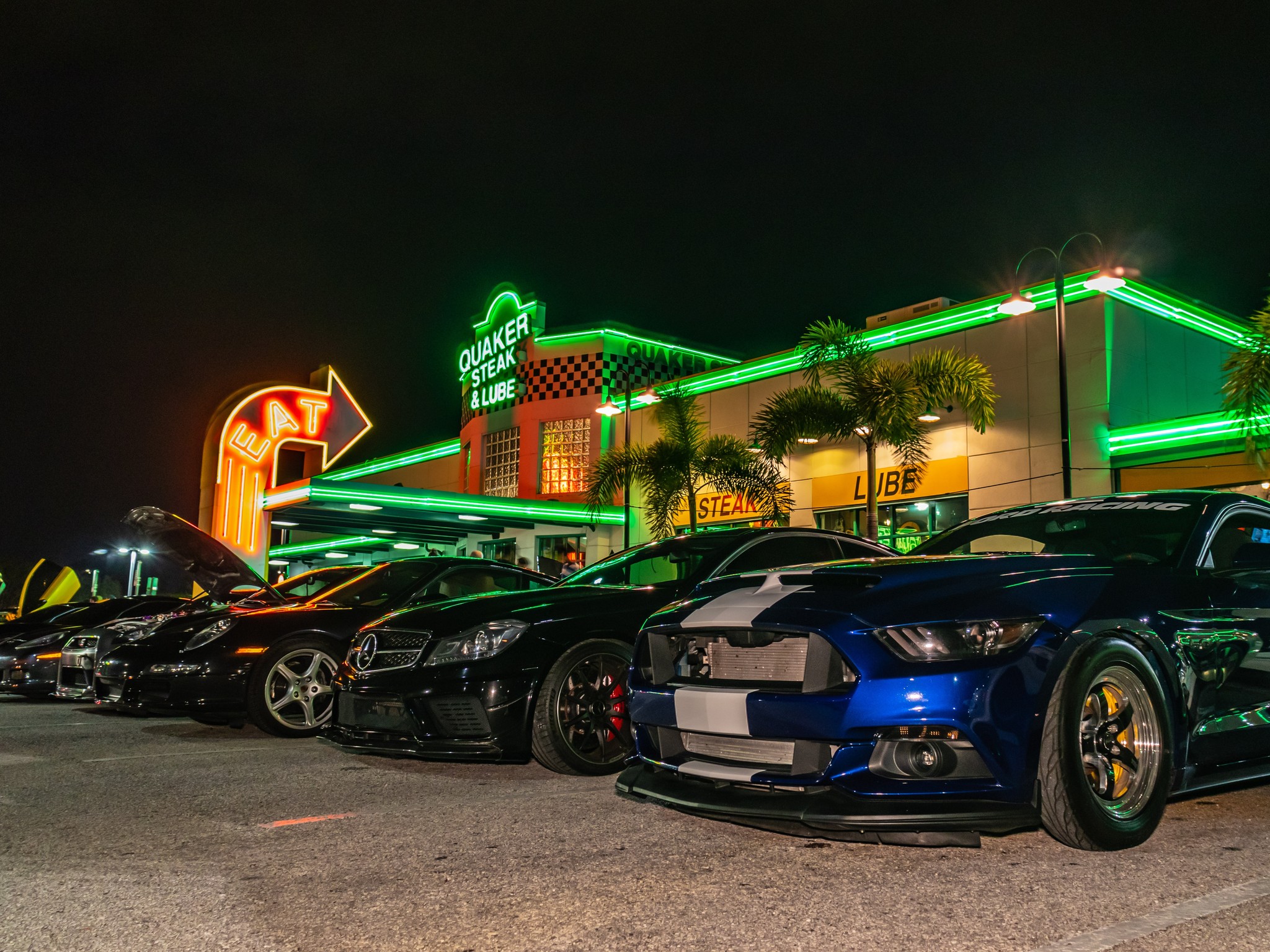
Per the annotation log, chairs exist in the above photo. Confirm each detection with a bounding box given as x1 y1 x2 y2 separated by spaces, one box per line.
439 574 464 597
469 574 497 594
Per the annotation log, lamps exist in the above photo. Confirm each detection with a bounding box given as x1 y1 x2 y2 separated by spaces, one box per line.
744 429 766 453
918 401 954 424
797 430 819 445
854 418 872 436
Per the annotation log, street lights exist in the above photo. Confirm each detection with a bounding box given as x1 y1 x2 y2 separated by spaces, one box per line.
996 232 1126 498
594 359 662 584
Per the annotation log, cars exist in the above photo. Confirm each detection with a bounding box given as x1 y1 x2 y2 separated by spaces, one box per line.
616 490 1270 853
315 527 906 778
0 503 564 738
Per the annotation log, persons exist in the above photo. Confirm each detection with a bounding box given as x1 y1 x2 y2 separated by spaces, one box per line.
90 596 98 603
428 548 441 556
470 550 484 559
516 557 528 567
